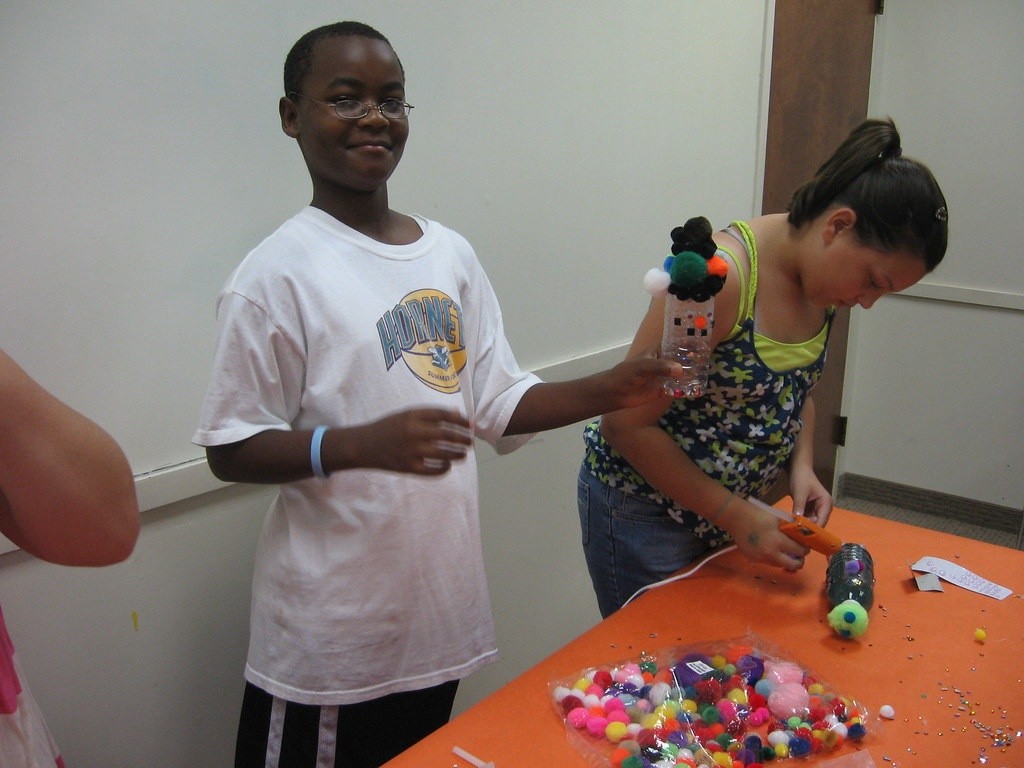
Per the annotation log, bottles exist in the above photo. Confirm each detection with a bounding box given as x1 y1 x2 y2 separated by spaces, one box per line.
826 543 874 638
660 289 714 398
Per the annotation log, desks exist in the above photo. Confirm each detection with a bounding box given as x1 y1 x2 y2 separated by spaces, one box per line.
375 495 1023 768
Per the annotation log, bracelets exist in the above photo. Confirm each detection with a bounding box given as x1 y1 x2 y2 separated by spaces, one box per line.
310 424 329 482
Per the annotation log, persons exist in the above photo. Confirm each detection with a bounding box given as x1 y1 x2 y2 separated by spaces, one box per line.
191 20 713 767
575 118 949 619
0 343 141 768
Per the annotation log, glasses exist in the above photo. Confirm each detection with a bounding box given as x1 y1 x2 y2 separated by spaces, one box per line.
287 91 414 119
